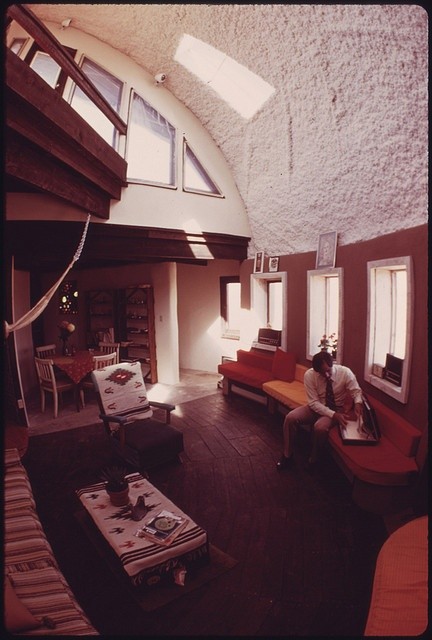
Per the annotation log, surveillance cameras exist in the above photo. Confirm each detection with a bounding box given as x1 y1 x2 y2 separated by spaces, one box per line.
60 16 73 30
153 73 170 86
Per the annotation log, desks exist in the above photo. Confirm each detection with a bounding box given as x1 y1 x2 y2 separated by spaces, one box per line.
43 348 118 410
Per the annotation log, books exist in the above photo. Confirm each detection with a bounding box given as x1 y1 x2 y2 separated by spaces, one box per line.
138 512 188 548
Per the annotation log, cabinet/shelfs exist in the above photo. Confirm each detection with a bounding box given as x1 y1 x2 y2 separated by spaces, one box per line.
90 283 157 385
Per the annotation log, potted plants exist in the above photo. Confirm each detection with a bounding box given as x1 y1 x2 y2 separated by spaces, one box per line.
104 469 132 506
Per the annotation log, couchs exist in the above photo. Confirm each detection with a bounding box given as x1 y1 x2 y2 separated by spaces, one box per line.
263 364 310 414
324 388 422 488
1 446 96 636
218 350 274 409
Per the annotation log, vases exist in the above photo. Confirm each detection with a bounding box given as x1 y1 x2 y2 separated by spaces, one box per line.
321 349 328 356
333 349 338 357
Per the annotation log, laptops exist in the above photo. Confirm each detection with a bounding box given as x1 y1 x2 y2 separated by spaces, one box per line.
339 396 381 446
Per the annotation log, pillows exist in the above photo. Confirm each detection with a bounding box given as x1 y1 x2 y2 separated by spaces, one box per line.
273 357 297 384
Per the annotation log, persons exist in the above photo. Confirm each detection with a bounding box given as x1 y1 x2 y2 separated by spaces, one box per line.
318 241 333 266
277 353 364 471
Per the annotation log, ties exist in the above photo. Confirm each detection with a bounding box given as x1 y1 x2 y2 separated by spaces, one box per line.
325 379 336 412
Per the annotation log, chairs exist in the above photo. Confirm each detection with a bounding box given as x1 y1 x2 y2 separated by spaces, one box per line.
101 343 120 351
38 345 55 356
92 361 185 477
35 358 82 419
93 354 117 367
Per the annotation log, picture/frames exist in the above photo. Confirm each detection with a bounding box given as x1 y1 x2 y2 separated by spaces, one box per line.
316 257 335 269
269 259 279 270
253 257 265 274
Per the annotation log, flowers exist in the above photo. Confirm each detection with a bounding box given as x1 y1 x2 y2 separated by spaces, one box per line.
332 338 339 347
319 336 331 347
